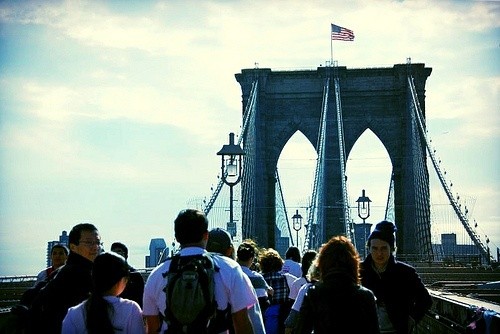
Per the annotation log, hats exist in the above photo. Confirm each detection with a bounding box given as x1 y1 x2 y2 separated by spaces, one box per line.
367 221 393 247
206 228 232 253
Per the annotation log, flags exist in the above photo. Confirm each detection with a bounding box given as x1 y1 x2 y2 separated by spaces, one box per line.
332 24 354 41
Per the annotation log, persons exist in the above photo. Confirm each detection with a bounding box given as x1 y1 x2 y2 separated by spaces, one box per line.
143 209 258 334
35 220 432 334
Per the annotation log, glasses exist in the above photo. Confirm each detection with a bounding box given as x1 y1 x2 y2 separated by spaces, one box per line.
79 238 103 248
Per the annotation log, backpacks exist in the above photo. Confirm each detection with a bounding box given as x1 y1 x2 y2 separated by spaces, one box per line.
12 267 60 334
162 252 224 334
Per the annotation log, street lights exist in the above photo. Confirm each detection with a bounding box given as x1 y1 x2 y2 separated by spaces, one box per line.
216 133 247 240
290 210 304 247
355 189 372 259
